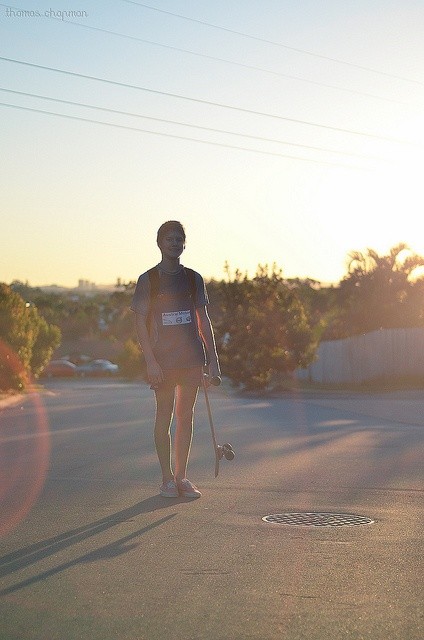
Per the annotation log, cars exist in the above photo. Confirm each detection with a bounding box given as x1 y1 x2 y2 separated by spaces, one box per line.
41 359 79 377
74 359 118 378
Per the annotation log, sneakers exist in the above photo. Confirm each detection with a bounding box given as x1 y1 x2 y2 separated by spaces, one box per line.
174 479 201 498
159 480 179 498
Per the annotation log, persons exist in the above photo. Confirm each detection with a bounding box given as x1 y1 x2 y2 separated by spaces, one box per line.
132 221 221 501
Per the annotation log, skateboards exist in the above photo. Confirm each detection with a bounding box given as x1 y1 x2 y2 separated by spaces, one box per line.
199 368 235 478
